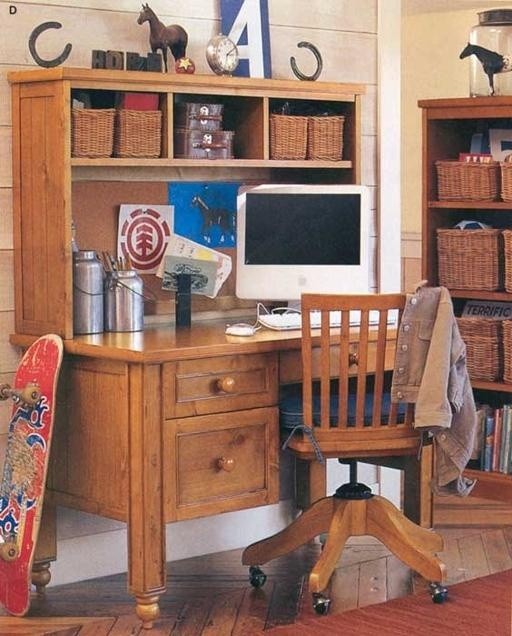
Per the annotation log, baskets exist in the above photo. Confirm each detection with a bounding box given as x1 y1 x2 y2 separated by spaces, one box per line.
113 107 164 158
269 113 308 160
307 112 345 161
71 107 117 158
434 158 512 385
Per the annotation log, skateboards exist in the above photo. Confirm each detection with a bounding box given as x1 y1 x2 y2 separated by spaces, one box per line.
1 333 64 618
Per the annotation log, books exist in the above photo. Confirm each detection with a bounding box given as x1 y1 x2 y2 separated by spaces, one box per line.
153 232 234 301
461 397 511 477
159 254 220 297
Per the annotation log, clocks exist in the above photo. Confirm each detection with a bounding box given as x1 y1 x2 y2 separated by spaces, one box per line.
206 35 241 75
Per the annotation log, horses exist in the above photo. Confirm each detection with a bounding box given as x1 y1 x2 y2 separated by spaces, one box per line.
188 196 237 246
136 2 190 75
458 42 512 97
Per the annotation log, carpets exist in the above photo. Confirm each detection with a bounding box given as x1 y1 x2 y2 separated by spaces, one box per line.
245 570 512 636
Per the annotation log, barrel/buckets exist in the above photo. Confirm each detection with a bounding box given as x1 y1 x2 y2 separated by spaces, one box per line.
103 268 159 334
71 250 122 334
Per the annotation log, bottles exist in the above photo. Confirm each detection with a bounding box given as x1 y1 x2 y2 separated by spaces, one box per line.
465 6 511 97
74 250 104 335
103 270 146 332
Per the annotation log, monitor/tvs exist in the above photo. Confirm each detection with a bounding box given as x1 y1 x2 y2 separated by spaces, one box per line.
236 183 370 301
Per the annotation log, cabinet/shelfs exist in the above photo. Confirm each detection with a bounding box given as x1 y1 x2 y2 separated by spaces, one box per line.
416 94 511 505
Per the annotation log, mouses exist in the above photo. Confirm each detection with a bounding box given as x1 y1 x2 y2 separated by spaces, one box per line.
225 322 255 336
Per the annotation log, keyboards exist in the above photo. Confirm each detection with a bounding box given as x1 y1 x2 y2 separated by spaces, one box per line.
258 308 399 330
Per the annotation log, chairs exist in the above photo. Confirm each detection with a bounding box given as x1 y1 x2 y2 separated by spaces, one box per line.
239 292 450 614
